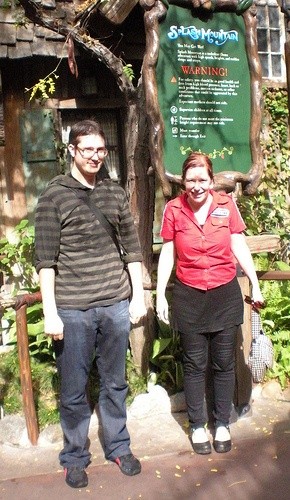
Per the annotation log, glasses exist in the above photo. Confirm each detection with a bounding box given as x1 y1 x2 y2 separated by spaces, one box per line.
76 146 108 159
244 295 263 308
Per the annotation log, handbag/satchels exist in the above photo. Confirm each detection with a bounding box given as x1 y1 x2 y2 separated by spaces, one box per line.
248 310 274 383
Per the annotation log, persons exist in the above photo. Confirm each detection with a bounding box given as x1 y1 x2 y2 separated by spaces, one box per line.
155 152 266 454
34 120 148 488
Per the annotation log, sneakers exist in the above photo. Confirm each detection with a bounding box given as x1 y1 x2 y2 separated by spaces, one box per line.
64 466 88 487
115 454 141 476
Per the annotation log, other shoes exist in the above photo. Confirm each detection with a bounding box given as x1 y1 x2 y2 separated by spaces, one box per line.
192 427 211 454
213 426 231 453
